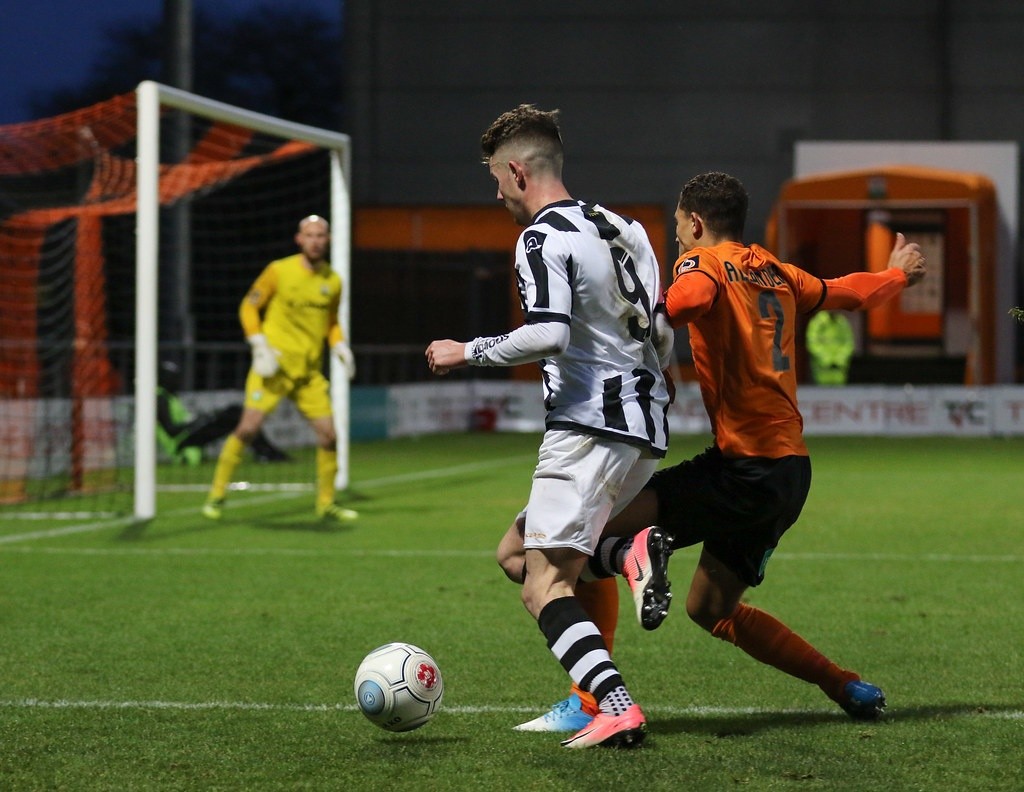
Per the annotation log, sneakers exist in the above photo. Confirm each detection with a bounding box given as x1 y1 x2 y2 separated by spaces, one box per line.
560 704 645 750
839 680 887 723
511 693 594 732
622 526 673 631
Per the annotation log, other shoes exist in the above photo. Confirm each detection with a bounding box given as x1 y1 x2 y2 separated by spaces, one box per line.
202 495 224 518
316 504 358 523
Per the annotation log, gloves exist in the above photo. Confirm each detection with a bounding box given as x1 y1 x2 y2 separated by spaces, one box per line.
249 335 282 379
335 343 355 377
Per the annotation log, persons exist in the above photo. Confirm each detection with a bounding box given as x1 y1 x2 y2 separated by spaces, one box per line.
156 361 294 465
804 306 856 384
421 104 675 751
511 173 928 734
201 214 362 522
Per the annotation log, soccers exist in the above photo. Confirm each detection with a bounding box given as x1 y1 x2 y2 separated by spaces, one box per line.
353 643 444 733
